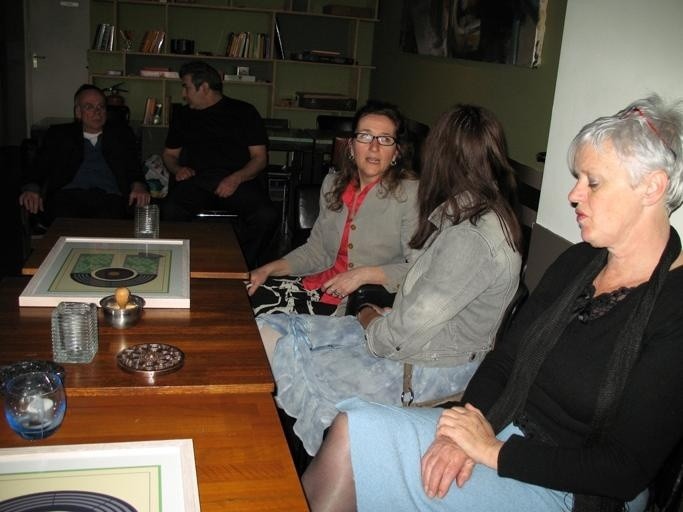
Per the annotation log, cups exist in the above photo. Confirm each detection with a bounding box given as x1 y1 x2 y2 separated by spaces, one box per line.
50 302 97 365
133 204 159 240
3 371 67 440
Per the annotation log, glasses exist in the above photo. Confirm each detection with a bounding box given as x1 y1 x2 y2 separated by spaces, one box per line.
616 105 676 160
82 104 105 113
352 133 397 146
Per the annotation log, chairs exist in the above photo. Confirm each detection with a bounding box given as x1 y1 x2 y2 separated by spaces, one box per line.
259 115 354 200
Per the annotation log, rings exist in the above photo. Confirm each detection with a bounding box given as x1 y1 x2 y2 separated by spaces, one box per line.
329 286 336 292
340 294 346 298
335 289 340 295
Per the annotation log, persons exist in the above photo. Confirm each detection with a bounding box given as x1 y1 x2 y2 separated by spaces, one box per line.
253 104 525 476
16 83 151 236
162 60 275 274
243 101 421 320
300 94 682 512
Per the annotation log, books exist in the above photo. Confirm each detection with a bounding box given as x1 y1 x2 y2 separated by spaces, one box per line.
140 95 156 123
91 22 167 56
226 29 268 60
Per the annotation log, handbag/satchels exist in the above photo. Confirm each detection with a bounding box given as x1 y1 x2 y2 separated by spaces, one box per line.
142 154 170 198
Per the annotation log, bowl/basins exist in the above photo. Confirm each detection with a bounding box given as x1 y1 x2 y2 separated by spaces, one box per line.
99 295 144 330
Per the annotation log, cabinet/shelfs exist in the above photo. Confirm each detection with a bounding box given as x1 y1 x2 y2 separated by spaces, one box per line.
86 6 383 204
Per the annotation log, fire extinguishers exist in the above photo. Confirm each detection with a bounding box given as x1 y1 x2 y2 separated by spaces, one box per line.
101 81 128 107
